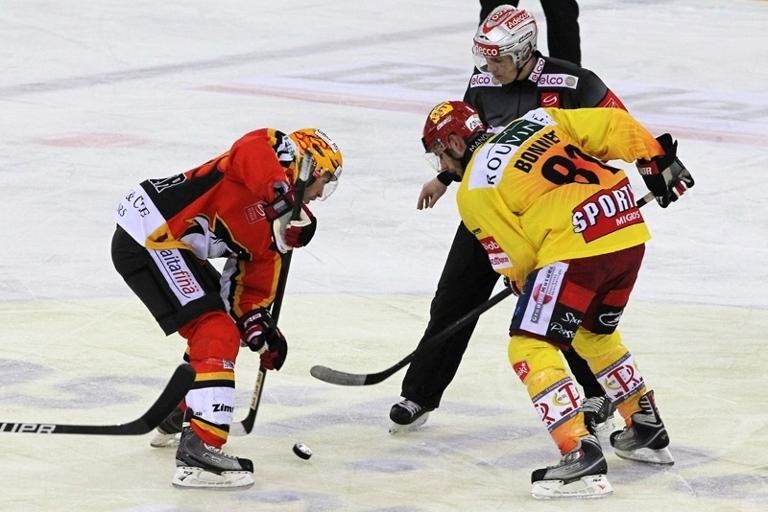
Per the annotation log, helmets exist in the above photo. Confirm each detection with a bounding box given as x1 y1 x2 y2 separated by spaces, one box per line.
472 4 537 66
289 128 343 178
422 101 484 154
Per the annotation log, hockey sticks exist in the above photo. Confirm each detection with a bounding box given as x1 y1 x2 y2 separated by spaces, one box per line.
310 192 655 385
228 149 313 436
0 364 195 434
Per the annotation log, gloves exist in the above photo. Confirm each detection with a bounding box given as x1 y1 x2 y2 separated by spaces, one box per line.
236 307 286 369
272 190 316 255
635 133 694 209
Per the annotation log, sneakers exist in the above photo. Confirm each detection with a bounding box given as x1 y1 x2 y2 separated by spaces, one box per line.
158 407 182 434
390 399 426 424
531 393 670 482
175 426 253 473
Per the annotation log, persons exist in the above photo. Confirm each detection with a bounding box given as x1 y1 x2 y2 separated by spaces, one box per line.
420 99 696 482
388 3 626 426
111 126 344 472
478 0 581 66
